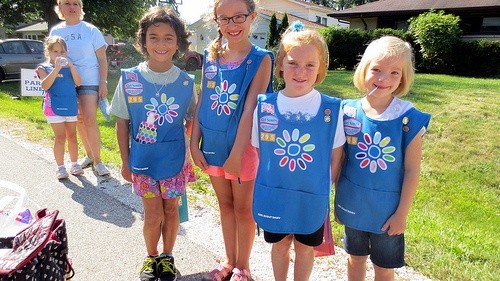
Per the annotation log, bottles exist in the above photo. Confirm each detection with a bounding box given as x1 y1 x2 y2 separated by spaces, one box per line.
56 56 69 68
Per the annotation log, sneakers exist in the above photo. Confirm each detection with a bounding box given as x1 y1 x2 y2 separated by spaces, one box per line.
156 253 178 281
55 166 69 179
80 156 93 169
138 256 158 281
93 161 110 177
69 162 85 175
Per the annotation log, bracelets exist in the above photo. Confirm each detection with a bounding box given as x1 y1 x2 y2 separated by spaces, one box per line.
99 80 109 85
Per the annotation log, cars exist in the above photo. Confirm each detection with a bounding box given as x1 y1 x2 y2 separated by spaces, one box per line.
107 43 128 66
0 38 48 83
175 50 202 72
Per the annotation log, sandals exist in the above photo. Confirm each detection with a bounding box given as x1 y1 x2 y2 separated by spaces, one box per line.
228 267 252 281
202 259 235 281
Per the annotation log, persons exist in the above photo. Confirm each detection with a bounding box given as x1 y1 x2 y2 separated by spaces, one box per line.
190 0 274 281
251 30 347 281
35 35 84 179
333 36 432 281
48 0 110 178
109 7 198 281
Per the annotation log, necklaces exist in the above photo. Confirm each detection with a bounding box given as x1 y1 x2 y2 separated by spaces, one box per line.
147 60 172 97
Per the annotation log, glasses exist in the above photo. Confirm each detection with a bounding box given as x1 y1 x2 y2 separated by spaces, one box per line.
214 12 251 26
